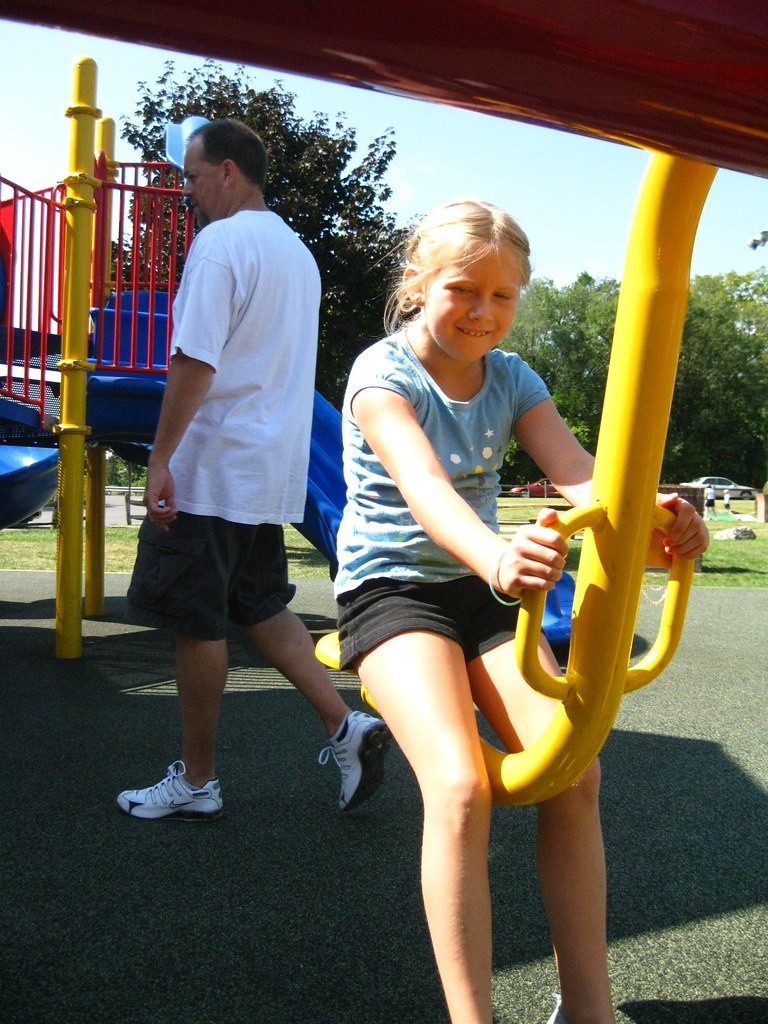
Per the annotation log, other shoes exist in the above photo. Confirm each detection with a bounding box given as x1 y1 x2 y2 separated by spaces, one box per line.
546 992 570 1024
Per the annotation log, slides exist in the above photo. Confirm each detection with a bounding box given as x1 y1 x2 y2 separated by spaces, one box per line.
84 304 578 646
1 443 62 533
111 442 154 468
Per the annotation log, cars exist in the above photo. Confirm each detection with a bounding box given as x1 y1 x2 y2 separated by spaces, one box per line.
509 478 561 498
678 477 758 501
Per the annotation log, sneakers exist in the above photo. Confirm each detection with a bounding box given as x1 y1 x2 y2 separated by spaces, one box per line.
317 710 392 812
115 761 223 822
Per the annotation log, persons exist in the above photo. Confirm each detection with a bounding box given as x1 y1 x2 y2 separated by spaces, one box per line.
115 118 390 823
335 201 710 1024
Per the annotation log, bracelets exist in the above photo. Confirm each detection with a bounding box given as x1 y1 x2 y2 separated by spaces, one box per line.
489 542 527 608
